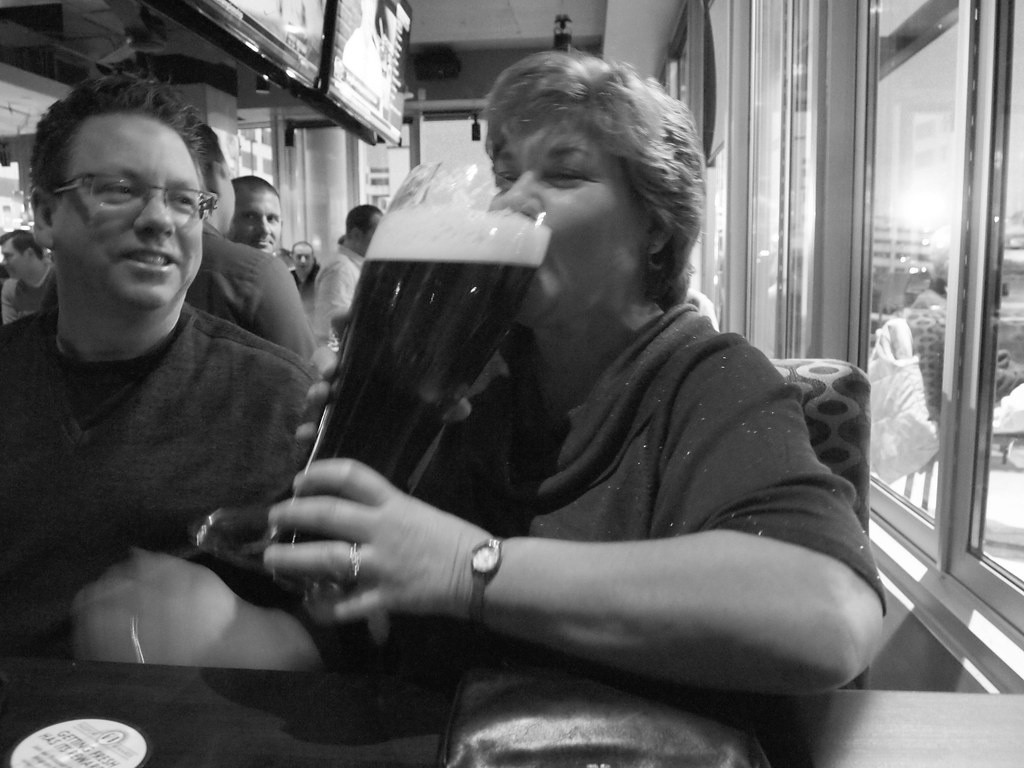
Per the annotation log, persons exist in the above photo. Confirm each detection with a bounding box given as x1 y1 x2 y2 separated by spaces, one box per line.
261 48 887 701
0 68 394 673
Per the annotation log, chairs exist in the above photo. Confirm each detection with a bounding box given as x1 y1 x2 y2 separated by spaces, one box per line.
870 316 940 512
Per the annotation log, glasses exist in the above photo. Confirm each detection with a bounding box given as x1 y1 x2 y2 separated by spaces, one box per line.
53 173 217 223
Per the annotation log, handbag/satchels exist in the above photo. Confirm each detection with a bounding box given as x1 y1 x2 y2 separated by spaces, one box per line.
438 666 771 768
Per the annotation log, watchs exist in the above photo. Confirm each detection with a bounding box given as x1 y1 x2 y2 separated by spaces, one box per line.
469 535 505 626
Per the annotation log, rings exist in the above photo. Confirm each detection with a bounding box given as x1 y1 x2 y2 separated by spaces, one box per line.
341 541 361 592
127 614 148 666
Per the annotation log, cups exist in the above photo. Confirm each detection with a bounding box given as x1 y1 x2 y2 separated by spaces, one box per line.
264 161 553 592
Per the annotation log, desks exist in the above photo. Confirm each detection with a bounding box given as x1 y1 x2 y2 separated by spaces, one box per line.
0 656 1024 768
